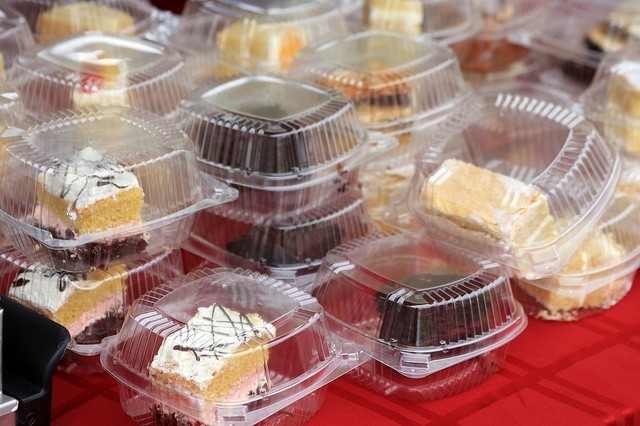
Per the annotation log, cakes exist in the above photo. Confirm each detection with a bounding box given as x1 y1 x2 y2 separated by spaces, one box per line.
180 106 312 221
20 52 129 109
587 9 637 54
365 2 421 35
520 223 631 318
36 2 132 38
234 152 244 153
222 214 345 277
10 265 125 343
605 59 639 156
309 63 414 123
376 273 490 375
214 24 304 82
35 146 150 271
420 157 555 256
152 304 270 426
